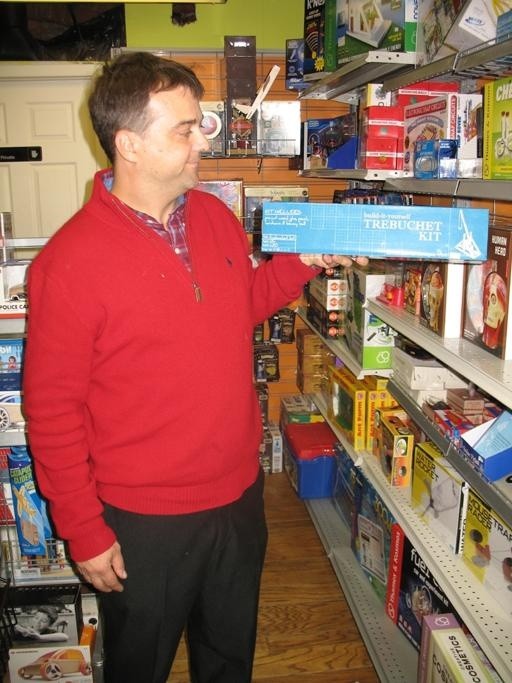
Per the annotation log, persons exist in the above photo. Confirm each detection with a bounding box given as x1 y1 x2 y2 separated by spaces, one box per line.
21 50 368 683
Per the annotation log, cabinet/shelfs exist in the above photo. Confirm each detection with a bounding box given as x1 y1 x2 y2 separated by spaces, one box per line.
297 32 512 682
126 49 352 427
